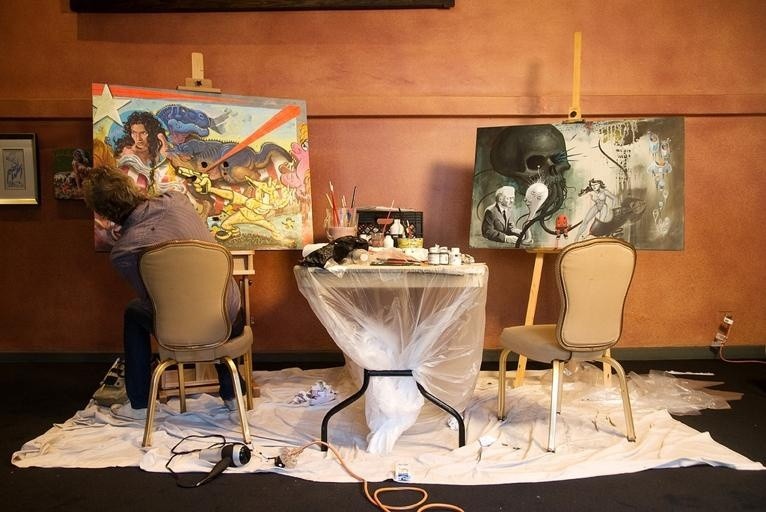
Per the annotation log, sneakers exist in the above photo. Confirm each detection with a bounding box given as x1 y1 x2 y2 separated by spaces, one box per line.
110 399 149 423
221 397 239 411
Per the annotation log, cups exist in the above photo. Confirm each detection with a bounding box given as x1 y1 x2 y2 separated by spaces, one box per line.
325 226 355 242
370 232 384 247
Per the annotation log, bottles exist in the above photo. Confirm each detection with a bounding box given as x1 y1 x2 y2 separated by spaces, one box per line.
383 234 395 248
347 249 369 267
390 218 405 248
428 246 462 264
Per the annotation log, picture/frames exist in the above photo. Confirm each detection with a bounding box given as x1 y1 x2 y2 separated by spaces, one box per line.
0 131 42 208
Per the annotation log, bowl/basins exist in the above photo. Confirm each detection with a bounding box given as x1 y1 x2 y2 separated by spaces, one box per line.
397 237 424 249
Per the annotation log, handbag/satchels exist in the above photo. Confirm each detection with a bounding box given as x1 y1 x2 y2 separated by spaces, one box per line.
303 236 368 267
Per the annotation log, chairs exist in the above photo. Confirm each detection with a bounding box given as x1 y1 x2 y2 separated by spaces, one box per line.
495 235 639 455
136 237 255 454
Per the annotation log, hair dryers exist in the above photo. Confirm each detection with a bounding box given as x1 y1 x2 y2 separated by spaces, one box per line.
194 442 251 488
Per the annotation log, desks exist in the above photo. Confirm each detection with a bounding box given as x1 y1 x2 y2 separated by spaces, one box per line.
292 243 490 455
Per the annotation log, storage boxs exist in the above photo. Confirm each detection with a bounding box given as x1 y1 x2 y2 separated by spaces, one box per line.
350 211 422 247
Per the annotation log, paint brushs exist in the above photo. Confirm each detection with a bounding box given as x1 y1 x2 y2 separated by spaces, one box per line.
399 207 413 239
382 199 395 234
324 176 357 227
370 258 428 266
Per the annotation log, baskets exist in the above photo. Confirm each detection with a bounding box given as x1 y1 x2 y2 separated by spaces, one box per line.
356 209 423 247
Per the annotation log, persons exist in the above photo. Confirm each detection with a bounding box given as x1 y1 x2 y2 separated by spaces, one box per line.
81 165 249 425
278 122 311 198
554 213 572 241
65 147 90 188
481 186 527 246
175 164 297 251
574 177 617 244
113 109 188 198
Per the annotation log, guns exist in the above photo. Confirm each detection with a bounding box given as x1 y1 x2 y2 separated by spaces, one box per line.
177 166 209 196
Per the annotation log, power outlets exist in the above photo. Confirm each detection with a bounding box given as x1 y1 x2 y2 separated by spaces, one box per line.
718 311 736 333
